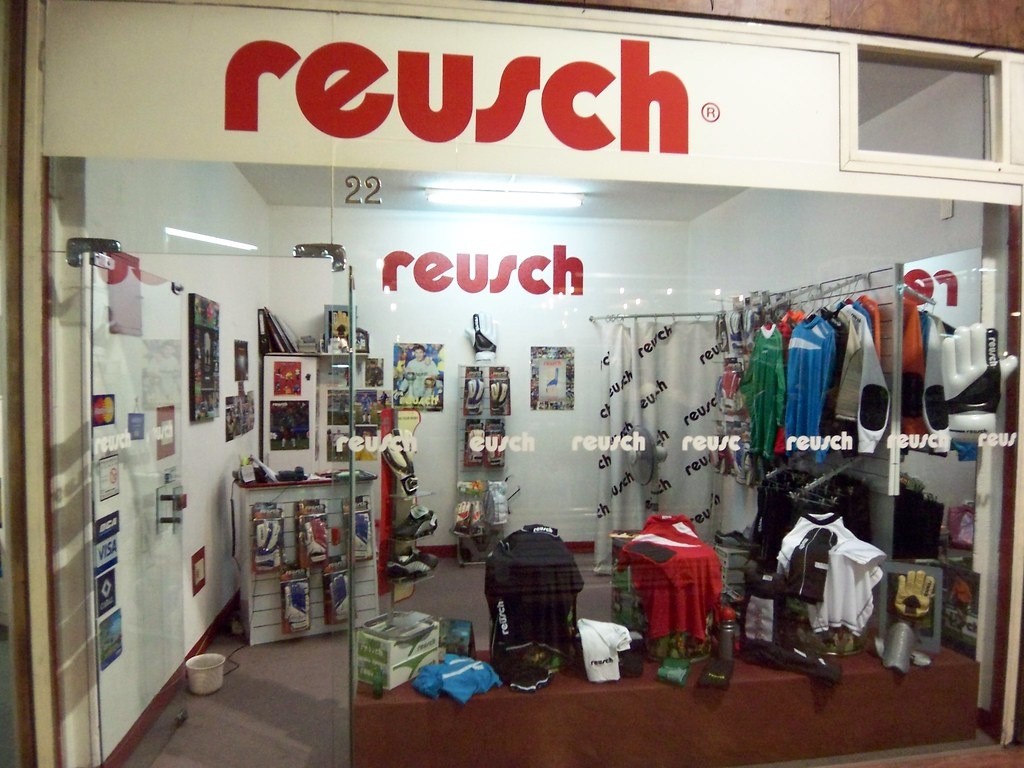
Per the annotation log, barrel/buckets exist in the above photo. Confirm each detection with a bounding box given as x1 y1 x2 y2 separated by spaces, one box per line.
186 653 226 695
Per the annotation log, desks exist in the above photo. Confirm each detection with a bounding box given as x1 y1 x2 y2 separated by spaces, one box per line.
353 628 981 768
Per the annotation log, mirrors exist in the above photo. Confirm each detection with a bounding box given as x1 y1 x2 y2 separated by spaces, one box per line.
889 246 983 571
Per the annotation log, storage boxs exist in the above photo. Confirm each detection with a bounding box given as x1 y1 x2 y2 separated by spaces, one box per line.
356 621 439 691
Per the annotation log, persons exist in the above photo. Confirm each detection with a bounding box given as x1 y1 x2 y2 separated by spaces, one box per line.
275 366 301 394
280 408 296 448
355 429 377 461
397 345 442 397
357 393 374 424
366 361 382 382
334 430 345 460
379 391 389 408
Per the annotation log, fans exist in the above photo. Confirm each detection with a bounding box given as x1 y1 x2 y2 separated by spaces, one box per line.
625 426 668 516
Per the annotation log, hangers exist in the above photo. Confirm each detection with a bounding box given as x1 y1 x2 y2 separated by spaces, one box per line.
760 273 864 328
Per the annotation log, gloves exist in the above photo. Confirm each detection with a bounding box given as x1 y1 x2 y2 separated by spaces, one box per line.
470 500 489 538
452 501 472 537
301 518 333 565
330 575 350 621
353 513 372 560
489 381 509 413
466 379 485 411
487 431 505 466
285 581 310 633
468 429 485 463
894 569 936 630
256 521 282 573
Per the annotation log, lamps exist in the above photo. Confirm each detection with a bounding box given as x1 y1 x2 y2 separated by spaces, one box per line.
423 188 584 209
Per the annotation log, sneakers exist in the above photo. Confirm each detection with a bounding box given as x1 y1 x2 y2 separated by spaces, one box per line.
391 508 442 540
385 546 439 582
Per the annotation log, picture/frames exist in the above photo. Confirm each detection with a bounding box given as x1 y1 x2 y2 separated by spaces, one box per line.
355 327 370 353
323 305 358 353
